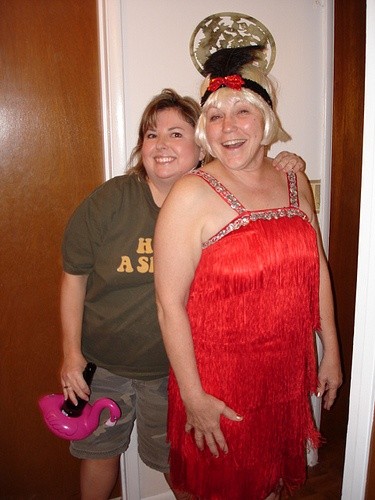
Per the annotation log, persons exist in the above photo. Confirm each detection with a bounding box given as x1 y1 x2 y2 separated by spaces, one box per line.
152 43 344 500
57 88 307 500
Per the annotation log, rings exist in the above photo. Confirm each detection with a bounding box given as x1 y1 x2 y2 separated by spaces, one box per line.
63 385 72 389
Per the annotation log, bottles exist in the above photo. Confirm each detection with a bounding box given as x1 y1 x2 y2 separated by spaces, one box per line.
61 362 97 418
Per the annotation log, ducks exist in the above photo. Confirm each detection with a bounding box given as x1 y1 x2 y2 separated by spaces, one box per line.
40 395 121 442
